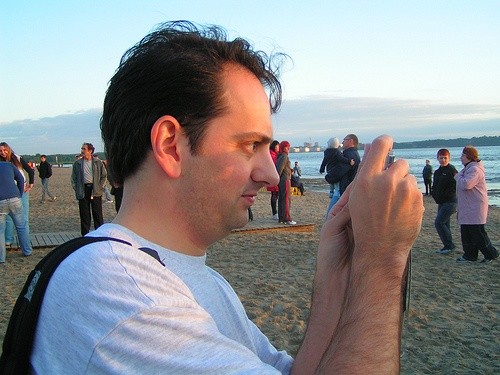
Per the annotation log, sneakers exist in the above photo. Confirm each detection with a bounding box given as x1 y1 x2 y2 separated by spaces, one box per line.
481 255 500 264
456 258 478 263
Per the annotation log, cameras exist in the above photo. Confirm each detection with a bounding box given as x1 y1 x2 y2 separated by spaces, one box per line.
384 152 394 172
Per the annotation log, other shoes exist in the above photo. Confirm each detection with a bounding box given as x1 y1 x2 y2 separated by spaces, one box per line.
40 201 45 203
301 194 305 196
436 247 458 255
50 197 56 201
279 221 296 225
273 214 279 219
329 193 333 198
5 243 11 248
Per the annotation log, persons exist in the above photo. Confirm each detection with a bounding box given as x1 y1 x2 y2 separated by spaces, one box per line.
0 142 35 246
71 143 107 236
326 134 360 221
291 169 304 196
36 154 57 203
275 141 296 225
92 155 113 203
27 161 35 171
319 138 355 197
293 162 301 178
423 160 432 196
107 167 124 213
0 20 425 375
266 140 279 215
431 149 459 253
454 146 500 263
0 162 32 262
